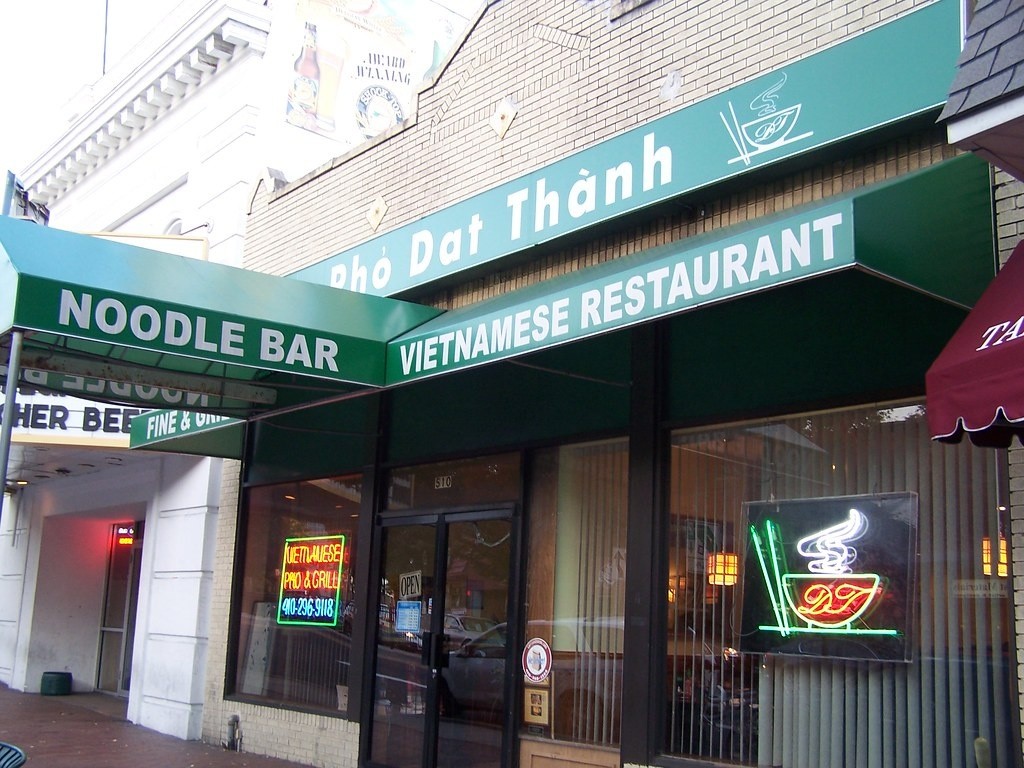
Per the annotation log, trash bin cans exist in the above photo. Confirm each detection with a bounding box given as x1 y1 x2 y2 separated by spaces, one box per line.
40 671 74 695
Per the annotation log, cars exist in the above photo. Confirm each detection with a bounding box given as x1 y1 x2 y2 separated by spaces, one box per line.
421 614 489 648
439 618 708 739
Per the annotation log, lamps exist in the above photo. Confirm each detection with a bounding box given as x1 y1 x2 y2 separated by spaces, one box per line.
708 547 738 586
